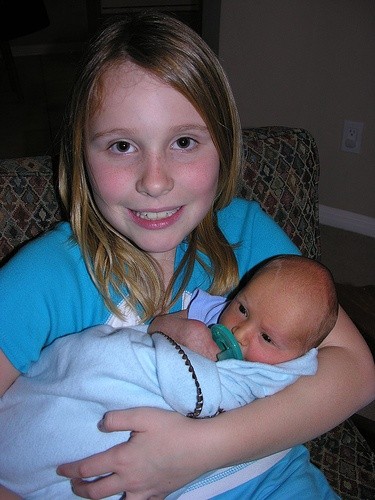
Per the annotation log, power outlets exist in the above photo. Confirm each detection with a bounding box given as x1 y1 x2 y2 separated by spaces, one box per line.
338 118 364 154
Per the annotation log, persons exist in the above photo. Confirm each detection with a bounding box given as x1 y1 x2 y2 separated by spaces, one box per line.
0 12 375 499
0 253 340 499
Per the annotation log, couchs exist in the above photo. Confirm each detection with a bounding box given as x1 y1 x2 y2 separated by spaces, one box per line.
0 126 375 500
